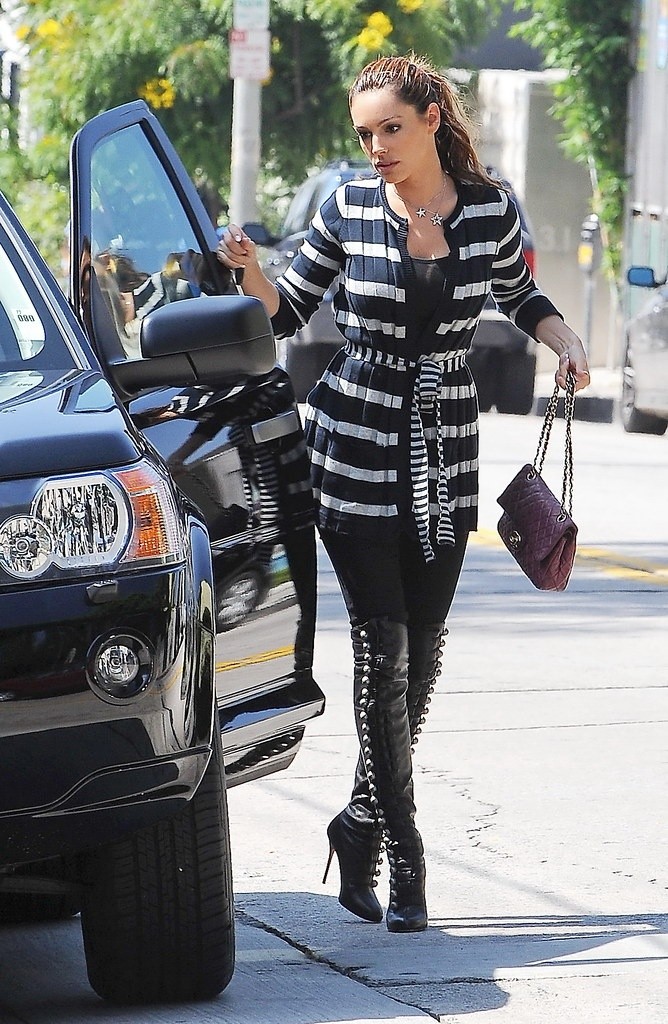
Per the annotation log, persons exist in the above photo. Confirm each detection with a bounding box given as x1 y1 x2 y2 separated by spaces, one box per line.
218 56 589 932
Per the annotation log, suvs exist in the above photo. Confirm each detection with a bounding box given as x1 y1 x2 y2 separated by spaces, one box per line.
245 151 536 423
0 98 327 1009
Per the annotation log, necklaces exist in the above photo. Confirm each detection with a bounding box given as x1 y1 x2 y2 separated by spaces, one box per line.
391 169 447 226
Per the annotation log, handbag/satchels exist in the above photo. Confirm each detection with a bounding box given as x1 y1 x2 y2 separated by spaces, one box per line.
497 371 579 592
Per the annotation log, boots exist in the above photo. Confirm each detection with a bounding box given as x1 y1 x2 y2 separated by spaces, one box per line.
352 618 427 933
322 621 449 921
